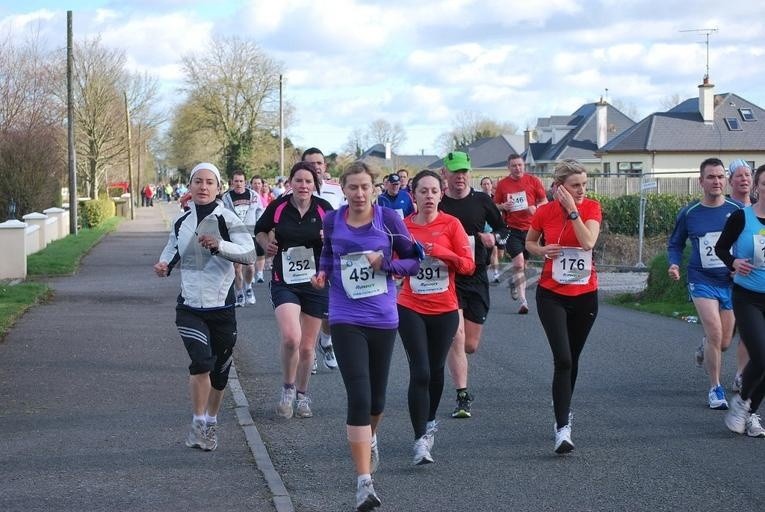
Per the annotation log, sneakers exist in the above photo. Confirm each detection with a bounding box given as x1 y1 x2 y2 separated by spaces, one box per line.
235 289 246 308
518 300 529 314
702 336 706 345
184 419 206 450
732 375 743 392
491 273 501 283
369 433 380 475
724 393 752 435
251 278 255 285
414 436 433 466
509 278 519 301
294 393 313 418
356 479 381 511
255 272 265 283
745 412 765 438
452 392 473 418
316 337 338 370
554 412 572 435
708 385 729 410
276 384 297 419
425 420 436 453
204 422 218 452
554 425 575 455
244 286 256 305
311 352 318 375
695 345 704 368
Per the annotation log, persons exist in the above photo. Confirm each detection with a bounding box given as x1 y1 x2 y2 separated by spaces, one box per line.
668 158 765 438
497 153 549 314
152 162 257 451
526 160 602 458
139 183 190 207
222 170 274 308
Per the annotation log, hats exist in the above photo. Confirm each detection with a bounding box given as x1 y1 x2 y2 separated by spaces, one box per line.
728 159 753 180
387 173 401 184
189 162 220 187
443 151 472 172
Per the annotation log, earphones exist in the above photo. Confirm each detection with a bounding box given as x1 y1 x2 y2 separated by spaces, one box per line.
372 190 375 193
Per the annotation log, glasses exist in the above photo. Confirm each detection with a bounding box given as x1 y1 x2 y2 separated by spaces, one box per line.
392 183 399 185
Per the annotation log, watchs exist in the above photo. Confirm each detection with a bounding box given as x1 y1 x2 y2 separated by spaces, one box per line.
568 210 580 220
